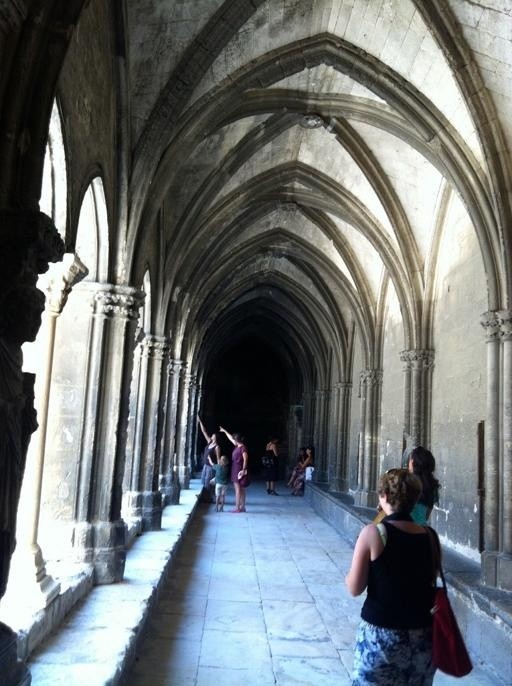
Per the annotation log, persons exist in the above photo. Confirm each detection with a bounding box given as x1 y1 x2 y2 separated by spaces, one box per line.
409 446 441 525
197 414 315 512
346 468 442 686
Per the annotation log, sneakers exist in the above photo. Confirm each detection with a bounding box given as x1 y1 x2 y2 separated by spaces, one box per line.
215 507 247 512
267 489 278 496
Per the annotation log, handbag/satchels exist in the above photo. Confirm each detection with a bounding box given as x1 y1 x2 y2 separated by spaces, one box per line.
430 587 473 678
306 467 315 482
239 474 251 488
262 456 275 468
